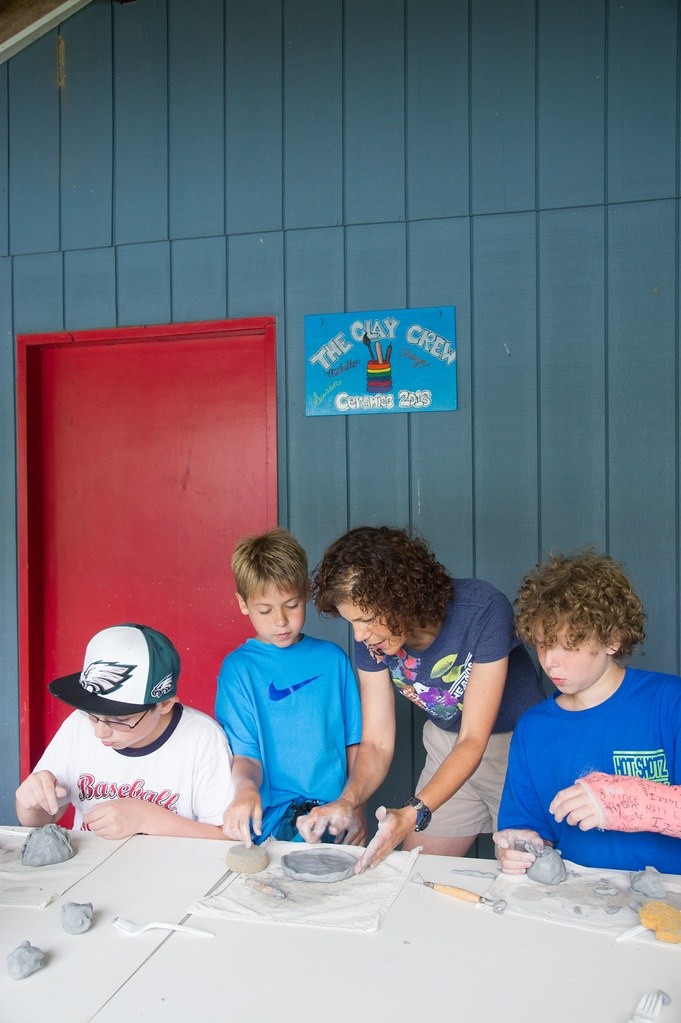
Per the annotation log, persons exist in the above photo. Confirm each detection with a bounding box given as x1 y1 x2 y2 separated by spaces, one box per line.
214 523 367 850
293 525 547 875
491 541 680 877
14 622 234 842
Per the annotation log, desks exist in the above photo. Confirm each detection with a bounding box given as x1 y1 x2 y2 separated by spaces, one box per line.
0 826 681 1021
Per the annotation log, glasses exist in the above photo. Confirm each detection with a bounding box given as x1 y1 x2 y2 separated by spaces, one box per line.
79 706 152 732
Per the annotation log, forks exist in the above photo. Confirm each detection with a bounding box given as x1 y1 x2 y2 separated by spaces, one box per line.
628 993 663 1023
112 917 216 939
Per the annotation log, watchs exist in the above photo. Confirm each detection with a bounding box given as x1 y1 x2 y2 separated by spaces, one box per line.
400 796 431 833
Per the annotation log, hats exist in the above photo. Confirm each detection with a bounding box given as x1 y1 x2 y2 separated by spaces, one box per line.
45 622 181 715
